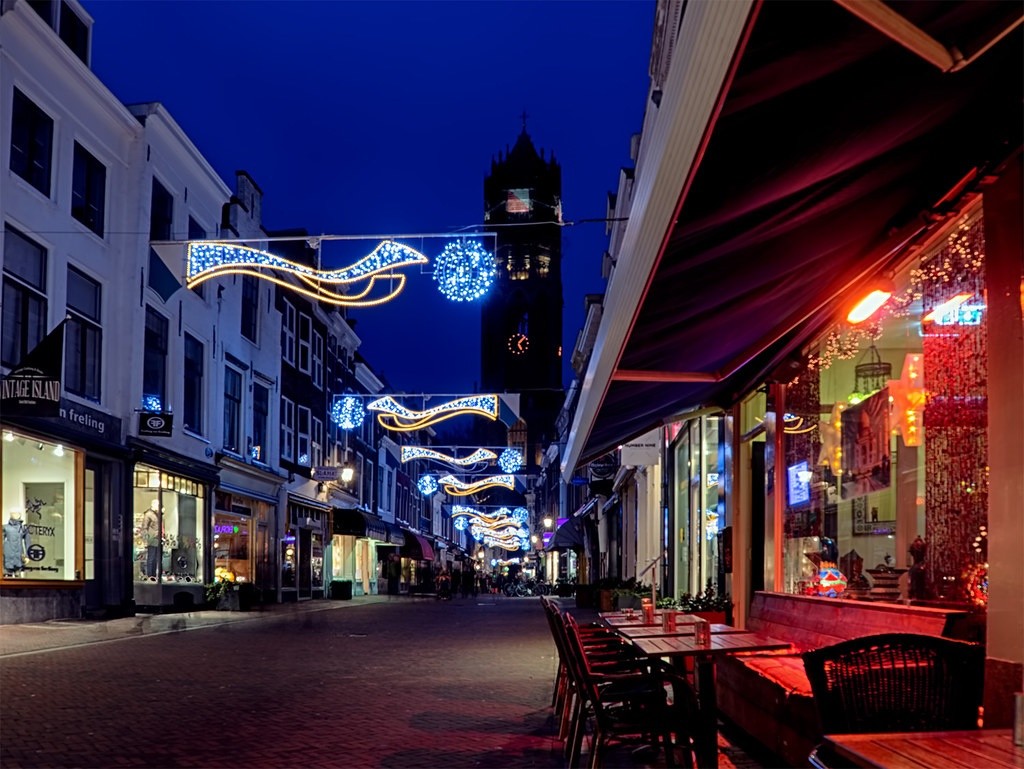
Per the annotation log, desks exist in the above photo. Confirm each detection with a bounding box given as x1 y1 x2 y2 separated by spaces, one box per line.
805 723 1024 769
592 604 792 769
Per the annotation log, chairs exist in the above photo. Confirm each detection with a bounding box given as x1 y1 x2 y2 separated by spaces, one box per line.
798 630 987 737
537 594 696 769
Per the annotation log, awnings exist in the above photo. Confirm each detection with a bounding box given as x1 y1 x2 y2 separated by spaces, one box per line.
552 3 1024 489
354 509 387 540
544 515 584 552
401 530 436 562
383 519 405 544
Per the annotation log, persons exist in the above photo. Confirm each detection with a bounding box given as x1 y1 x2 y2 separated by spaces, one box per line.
884 555 894 567
909 540 933 605
451 556 538 598
139 499 165 582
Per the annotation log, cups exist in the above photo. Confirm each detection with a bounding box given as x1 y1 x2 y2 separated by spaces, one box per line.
641 598 650 609
662 611 676 632
642 604 654 624
695 620 711 645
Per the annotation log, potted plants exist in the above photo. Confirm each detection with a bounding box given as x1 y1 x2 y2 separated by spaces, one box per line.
202 576 252 611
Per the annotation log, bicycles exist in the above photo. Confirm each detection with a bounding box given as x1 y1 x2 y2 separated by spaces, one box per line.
434 574 546 599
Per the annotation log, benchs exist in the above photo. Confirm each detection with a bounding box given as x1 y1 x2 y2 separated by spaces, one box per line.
695 588 969 769
